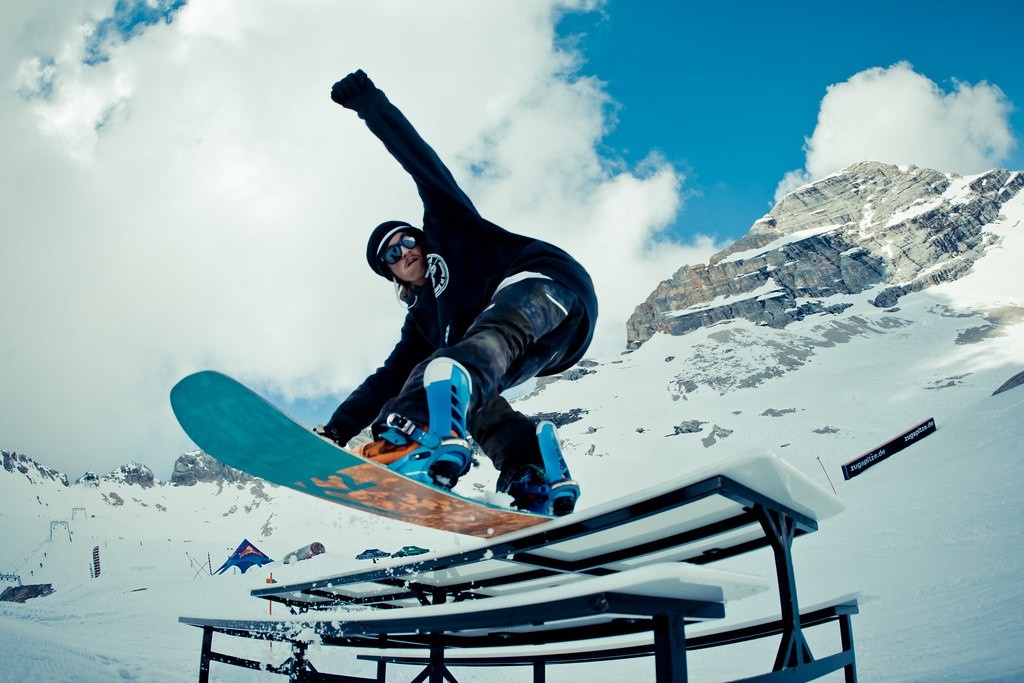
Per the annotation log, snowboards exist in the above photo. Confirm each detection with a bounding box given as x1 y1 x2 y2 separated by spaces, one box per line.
168 371 560 540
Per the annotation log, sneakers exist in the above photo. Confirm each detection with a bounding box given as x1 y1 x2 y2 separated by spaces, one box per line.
349 356 480 496
493 419 581 519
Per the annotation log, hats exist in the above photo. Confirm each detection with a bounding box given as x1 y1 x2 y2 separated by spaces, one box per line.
365 220 424 283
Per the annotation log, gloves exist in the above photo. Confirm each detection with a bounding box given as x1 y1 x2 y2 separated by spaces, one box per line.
330 69 375 112
313 426 348 450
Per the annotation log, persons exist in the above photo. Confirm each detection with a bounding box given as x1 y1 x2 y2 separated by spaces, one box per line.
309 68 600 517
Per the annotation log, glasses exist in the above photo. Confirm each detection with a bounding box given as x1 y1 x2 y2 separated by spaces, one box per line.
379 230 418 266
